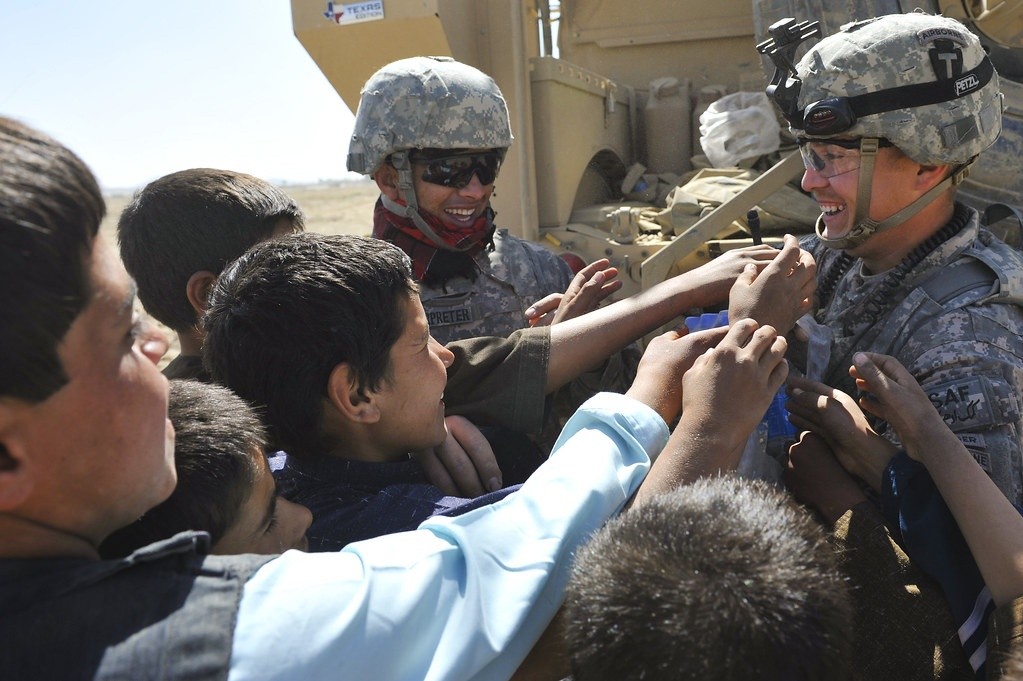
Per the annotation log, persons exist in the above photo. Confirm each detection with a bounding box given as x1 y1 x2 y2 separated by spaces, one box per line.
521 256 622 334
164 379 314 554
110 171 783 414
840 335 1023 681
202 231 815 546
0 111 728 681
732 8 1023 623
550 477 848 679
339 58 574 343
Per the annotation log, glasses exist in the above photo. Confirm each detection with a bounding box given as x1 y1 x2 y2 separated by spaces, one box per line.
384 151 502 188
799 134 895 176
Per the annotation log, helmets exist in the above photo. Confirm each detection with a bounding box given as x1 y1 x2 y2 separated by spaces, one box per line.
784 11 1005 165
346 55 515 175
